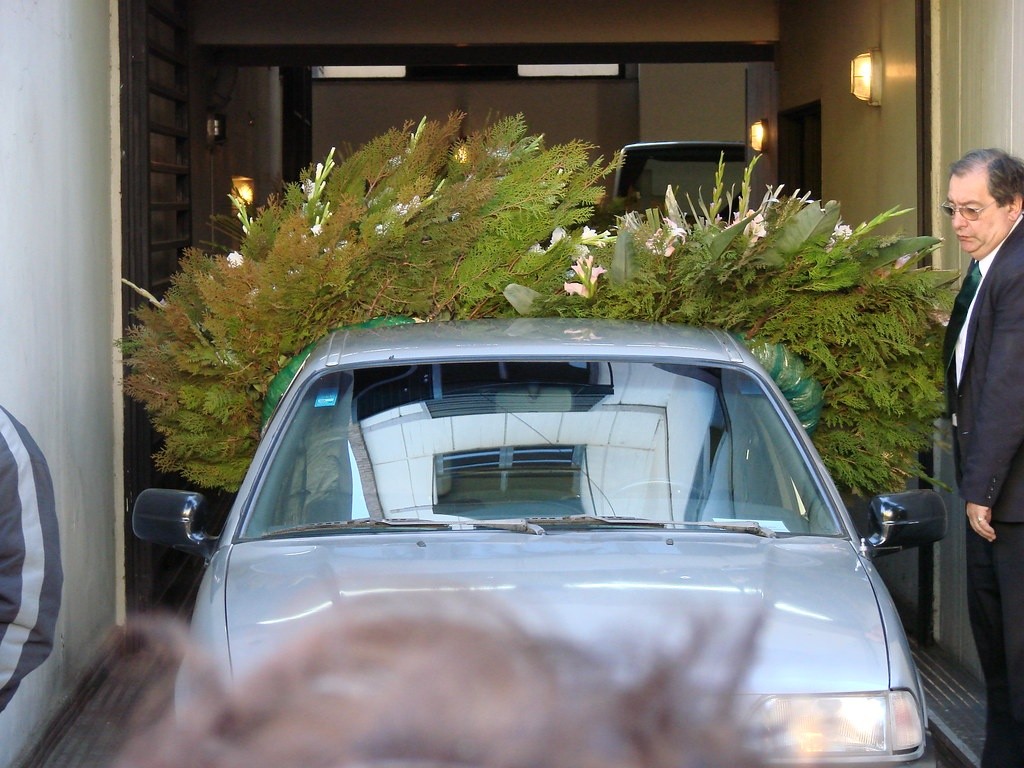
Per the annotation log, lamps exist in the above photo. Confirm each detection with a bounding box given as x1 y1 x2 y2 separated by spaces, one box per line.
750 119 769 152
850 47 882 107
231 176 254 217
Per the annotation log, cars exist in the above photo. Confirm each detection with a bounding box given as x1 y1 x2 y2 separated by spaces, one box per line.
131 316 953 768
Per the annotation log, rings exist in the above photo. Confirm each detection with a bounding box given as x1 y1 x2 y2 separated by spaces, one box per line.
977 517 986 522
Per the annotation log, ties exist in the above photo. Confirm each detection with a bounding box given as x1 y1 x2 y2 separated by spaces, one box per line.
944 261 982 416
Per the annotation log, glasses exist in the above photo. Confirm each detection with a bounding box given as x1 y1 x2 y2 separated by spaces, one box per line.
940 199 997 220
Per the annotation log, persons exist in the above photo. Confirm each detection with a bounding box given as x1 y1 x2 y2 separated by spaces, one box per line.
941 148 1024 768
